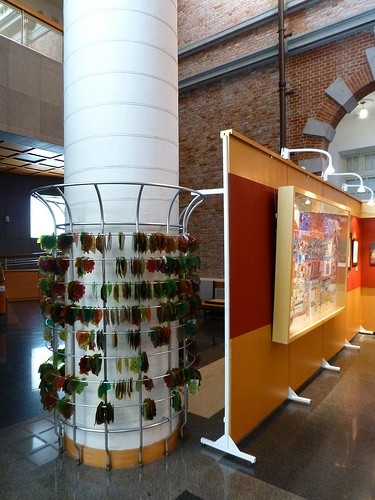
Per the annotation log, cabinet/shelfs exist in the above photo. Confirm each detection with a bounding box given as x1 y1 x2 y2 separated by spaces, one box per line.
4 271 48 301
29 183 204 472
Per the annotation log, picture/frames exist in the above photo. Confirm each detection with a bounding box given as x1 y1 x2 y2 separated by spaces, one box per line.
352 238 359 267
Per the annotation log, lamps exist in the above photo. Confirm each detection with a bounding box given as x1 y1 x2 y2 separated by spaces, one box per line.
281 147 335 175
360 102 369 119
323 173 365 192
344 184 374 207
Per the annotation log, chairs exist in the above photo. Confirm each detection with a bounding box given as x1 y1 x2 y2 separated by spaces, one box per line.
203 281 224 346
